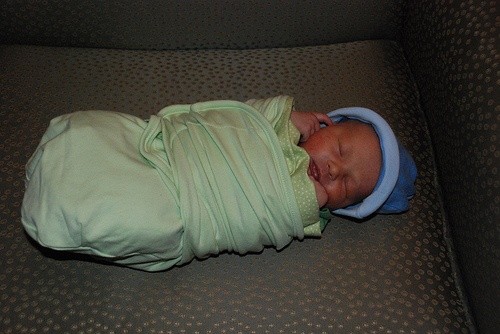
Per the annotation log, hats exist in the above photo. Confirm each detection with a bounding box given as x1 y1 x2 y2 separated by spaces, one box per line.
318 107 418 220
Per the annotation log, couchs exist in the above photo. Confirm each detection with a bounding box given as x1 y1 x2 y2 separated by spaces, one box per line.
0 0 500 334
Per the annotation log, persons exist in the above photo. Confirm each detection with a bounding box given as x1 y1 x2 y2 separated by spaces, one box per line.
19 94 419 275
